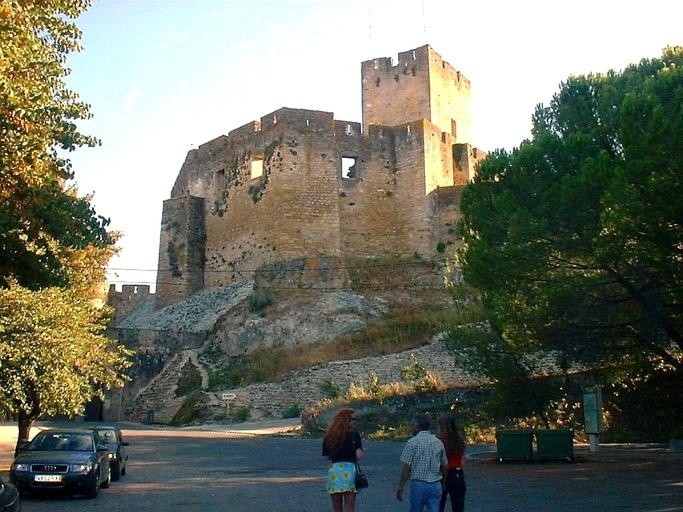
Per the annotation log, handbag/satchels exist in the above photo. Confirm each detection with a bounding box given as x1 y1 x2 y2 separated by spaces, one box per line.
356 472 368 489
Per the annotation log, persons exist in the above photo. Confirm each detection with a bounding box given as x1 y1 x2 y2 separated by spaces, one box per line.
394 414 448 512
320 407 366 512
435 416 467 511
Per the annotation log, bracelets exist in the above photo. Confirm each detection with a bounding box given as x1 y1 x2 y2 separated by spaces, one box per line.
397 488 404 492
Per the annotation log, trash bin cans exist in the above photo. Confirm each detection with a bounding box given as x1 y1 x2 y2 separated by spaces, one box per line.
536 429 574 464
495 430 533 464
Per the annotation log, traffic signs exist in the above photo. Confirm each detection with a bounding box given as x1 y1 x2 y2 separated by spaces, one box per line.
222 393 236 400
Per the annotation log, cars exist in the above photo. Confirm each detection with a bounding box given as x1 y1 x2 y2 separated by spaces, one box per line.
1 478 19 511
9 428 110 497
90 425 130 480
